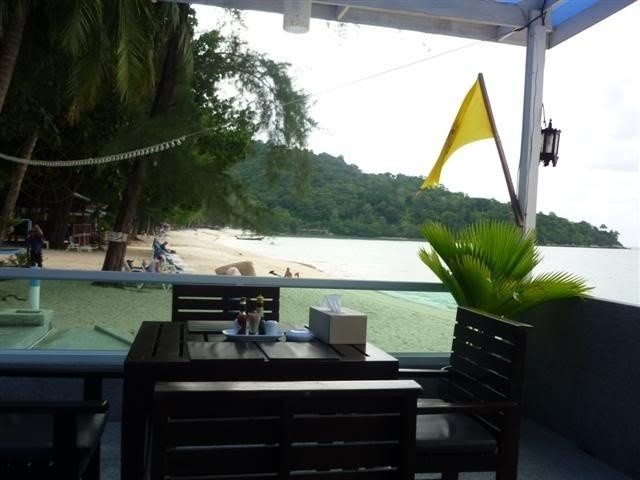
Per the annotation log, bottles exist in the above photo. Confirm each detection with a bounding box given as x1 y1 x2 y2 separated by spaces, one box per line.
237 296 248 334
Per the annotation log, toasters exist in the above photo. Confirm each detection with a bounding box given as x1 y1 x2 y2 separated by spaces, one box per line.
255 294 265 333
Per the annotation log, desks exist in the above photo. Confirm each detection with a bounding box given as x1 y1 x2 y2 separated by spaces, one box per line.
119 318 403 477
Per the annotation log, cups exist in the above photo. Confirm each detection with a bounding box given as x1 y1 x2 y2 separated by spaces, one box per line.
247 312 262 336
266 320 279 335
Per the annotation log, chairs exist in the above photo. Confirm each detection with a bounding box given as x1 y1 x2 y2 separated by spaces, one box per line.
139 376 426 479
343 305 535 479
1 394 113 480
169 280 282 331
124 258 182 290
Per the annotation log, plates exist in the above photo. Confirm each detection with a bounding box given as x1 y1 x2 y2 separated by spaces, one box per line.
221 329 284 342
285 331 314 342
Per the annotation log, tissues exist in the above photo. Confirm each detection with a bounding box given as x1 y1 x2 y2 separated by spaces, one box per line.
308 294 368 345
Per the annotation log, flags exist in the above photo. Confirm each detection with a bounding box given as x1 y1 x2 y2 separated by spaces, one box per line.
420 79 494 190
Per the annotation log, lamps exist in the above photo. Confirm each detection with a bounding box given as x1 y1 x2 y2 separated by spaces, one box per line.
538 115 562 169
282 0 313 35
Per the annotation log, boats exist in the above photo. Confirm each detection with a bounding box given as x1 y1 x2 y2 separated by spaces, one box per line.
235 229 264 241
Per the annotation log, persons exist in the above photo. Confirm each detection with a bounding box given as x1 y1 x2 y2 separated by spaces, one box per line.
26 224 44 268
149 241 170 273
